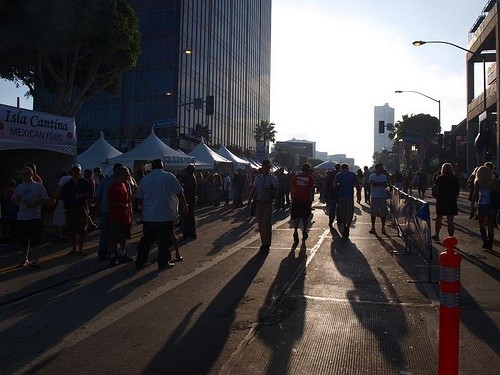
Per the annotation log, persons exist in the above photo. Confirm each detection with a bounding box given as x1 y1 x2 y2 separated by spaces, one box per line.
195 162 500 251
136 159 197 270
0 160 48 269
51 163 101 259
95 164 138 267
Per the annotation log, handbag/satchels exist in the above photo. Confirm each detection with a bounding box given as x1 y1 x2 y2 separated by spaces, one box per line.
385 192 392 199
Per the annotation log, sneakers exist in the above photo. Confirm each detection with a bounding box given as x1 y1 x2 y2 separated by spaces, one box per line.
119 254 133 262
109 256 119 266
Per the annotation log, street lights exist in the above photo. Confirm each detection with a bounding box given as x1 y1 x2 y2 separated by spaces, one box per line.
167 92 192 145
395 90 440 138
163 48 191 147
412 40 487 112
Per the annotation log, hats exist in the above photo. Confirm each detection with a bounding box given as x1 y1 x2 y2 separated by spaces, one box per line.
71 162 82 171
301 163 311 169
374 162 383 169
260 160 272 167
441 163 454 173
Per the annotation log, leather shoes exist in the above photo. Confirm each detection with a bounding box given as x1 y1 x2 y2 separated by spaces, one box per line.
135 259 143 270
159 260 176 270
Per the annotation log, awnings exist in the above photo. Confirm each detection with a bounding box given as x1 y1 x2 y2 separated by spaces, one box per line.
313 160 335 169
61 126 288 182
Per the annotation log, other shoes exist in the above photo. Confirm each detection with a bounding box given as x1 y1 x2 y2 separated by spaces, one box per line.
343 224 350 232
302 233 309 241
469 214 478 220
182 233 197 240
336 219 341 226
311 208 315 210
214 203 220 207
260 242 271 251
245 203 250 207
432 234 440 241
328 221 333 228
175 253 184 261
365 199 370 203
72 248 85 257
419 196 421 199
369 227 376 234
422 194 424 200
98 255 106 261
21 258 29 267
223 203 228 206
29 262 40 269
482 240 493 250
293 232 299 242
356 199 360 203
285 203 290 207
381 228 387 234
